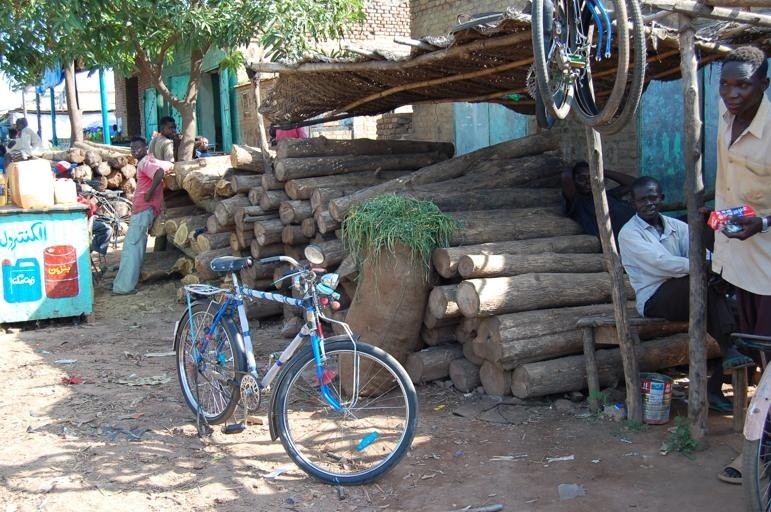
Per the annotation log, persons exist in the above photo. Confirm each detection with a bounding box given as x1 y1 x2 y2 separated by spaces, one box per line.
276 128 308 139
620 174 759 415
56 160 112 277
558 160 643 243
111 135 172 297
704 43 771 487
113 125 119 137
4 118 46 160
195 135 212 158
147 117 177 163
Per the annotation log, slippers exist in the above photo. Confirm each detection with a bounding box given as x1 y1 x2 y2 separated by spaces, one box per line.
709 401 735 415
721 353 756 375
717 453 768 483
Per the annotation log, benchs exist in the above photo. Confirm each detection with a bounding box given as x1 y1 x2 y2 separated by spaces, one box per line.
575 315 669 422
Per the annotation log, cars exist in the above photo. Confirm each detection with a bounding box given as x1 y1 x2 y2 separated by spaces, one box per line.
83 119 116 137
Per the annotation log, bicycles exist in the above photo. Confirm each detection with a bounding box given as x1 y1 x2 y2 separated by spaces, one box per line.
173 244 418 485
77 178 133 248
743 359 771 512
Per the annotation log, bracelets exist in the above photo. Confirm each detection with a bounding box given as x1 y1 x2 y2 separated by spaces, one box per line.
761 216 769 233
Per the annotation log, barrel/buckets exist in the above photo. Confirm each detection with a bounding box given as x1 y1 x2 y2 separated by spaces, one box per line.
2 258 42 303
43 246 79 298
639 370 674 424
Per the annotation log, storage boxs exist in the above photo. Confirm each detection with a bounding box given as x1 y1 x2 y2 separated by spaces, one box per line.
9 158 55 209
